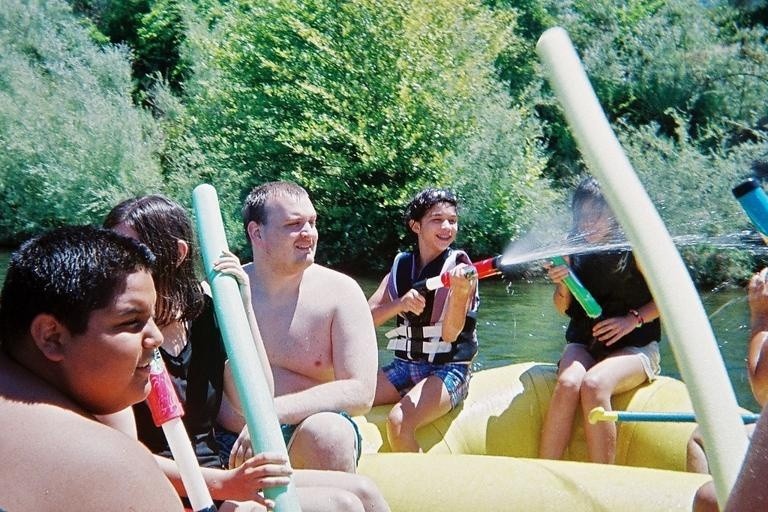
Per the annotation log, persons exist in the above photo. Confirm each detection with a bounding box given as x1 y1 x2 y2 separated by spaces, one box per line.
154 178 381 475
99 193 392 512
684 246 768 512
536 173 662 464
367 185 480 453
0 222 189 512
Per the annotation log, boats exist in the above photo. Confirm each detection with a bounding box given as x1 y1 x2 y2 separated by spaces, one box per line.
350 362 759 512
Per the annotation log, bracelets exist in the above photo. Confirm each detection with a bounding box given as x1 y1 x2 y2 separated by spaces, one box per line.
628 304 645 331
